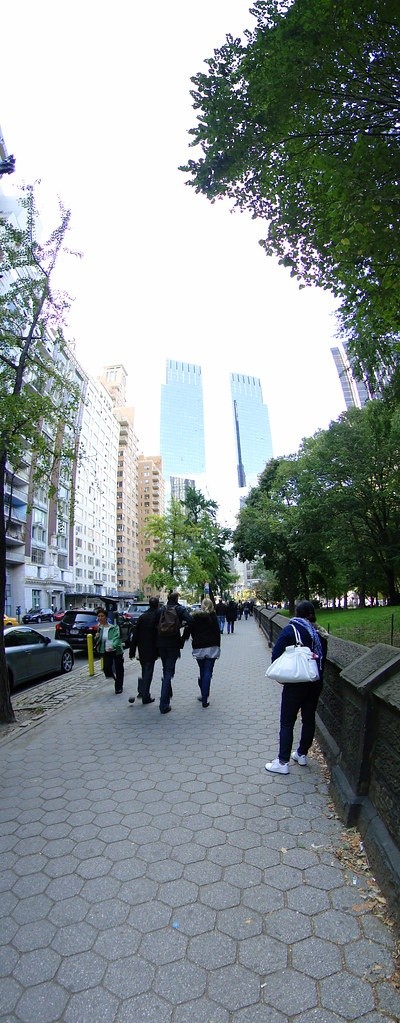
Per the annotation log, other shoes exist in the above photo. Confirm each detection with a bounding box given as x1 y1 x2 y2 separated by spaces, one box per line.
116 689 123 693
197 696 202 701
143 698 155 704
203 702 211 708
161 706 171 714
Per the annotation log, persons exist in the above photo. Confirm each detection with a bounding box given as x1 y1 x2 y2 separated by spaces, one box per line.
217 599 257 634
154 594 191 713
94 609 124 694
129 597 164 704
179 598 220 708
264 601 327 774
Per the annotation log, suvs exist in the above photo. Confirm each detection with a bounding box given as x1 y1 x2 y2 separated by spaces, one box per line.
54 608 136 652
124 601 150 622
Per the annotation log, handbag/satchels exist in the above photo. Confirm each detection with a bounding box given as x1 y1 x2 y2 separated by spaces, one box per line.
265 625 321 683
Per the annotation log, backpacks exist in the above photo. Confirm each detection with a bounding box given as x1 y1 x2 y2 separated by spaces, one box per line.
159 605 180 634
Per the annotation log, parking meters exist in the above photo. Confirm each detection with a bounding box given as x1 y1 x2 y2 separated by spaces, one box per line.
15 606 21 625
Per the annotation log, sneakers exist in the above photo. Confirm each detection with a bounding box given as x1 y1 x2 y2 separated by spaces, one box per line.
292 752 307 765
265 759 289 775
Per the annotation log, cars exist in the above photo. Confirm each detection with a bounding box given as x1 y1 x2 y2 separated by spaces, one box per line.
4 614 20 627
21 607 55 624
3 624 75 691
54 610 67 620
191 604 202 611
